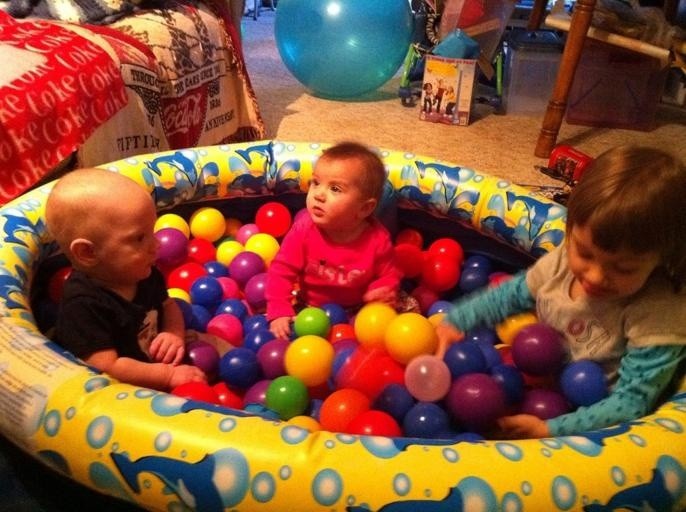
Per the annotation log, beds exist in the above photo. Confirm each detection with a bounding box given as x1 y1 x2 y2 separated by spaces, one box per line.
1 0 244 206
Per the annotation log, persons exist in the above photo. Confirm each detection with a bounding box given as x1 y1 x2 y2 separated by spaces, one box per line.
430 143 686 438
42 167 209 391
433 78 446 114
445 85 457 117
264 142 403 341
422 81 434 111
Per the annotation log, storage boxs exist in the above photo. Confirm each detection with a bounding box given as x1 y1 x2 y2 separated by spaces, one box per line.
565 35 676 131
501 28 565 113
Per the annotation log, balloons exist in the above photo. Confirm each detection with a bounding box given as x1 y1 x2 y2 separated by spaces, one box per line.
273 0 413 99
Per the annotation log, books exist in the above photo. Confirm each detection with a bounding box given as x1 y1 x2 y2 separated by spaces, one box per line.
418 54 478 126
